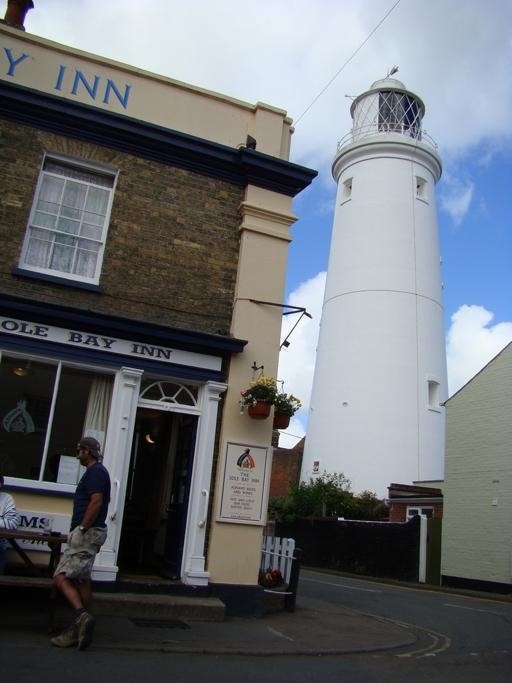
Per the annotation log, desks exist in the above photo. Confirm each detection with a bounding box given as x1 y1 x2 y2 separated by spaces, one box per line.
0 526 69 543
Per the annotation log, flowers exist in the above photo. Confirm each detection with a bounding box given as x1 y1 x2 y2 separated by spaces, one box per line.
239 374 301 413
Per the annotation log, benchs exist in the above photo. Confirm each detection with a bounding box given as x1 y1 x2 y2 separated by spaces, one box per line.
0 561 57 632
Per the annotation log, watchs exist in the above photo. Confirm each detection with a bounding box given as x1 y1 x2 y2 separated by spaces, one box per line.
79 524 86 533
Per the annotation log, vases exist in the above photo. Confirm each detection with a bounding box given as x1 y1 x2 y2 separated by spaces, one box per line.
273 411 293 428
249 399 271 418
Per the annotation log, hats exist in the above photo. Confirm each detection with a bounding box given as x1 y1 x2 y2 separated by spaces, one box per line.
77 436 105 460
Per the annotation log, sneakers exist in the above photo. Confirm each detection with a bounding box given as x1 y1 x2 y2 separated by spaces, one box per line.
49 625 79 648
71 611 95 651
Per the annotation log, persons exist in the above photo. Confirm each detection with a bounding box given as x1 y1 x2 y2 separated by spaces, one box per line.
51 436 111 650
0 473 20 570
